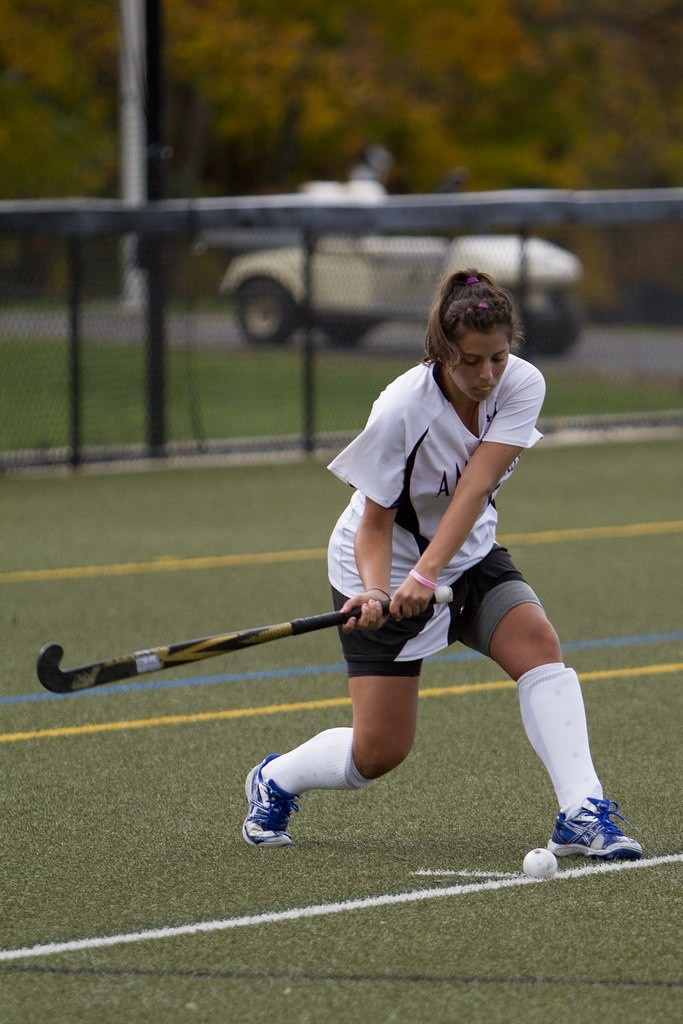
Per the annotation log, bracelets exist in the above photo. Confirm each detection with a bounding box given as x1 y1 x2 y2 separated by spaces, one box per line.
365 588 391 600
409 569 437 590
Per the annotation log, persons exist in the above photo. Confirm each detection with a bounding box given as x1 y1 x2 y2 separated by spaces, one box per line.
243 268 644 862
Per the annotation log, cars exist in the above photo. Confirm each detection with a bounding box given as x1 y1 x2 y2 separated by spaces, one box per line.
218 176 585 355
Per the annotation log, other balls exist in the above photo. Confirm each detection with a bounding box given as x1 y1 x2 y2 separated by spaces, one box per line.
521 847 559 879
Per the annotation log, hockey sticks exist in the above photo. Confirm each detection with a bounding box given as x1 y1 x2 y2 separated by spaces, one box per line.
34 584 454 695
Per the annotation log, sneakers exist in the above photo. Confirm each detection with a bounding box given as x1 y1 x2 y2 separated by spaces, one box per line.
549 800 644 862
243 754 295 848
